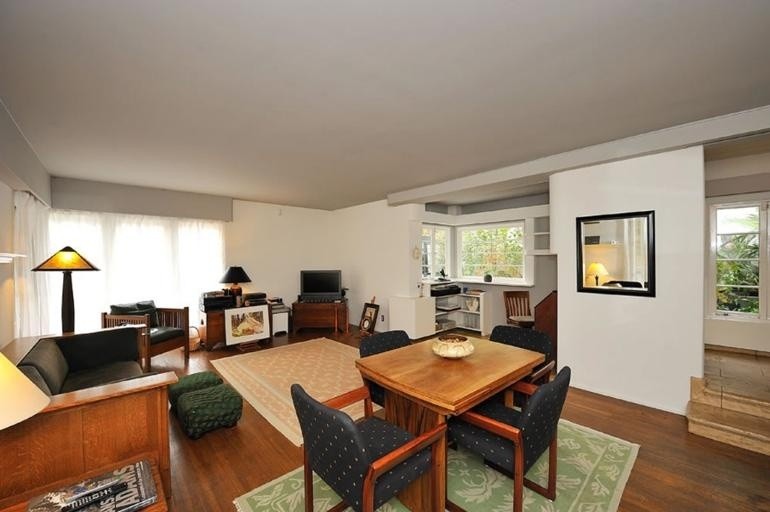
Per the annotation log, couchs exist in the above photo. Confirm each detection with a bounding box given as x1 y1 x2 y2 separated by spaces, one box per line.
1 323 170 500
602 280 648 288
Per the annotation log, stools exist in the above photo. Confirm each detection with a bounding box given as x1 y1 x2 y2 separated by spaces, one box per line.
170 369 224 415
176 383 244 439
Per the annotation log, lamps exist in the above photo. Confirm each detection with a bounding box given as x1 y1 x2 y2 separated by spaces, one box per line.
218 265 253 298
28 240 101 334
587 262 608 287
0 346 49 434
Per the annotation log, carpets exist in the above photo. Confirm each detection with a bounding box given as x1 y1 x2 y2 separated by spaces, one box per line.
229 419 642 510
208 336 388 449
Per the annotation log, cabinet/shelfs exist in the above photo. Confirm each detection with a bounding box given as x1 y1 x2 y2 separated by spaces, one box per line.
290 297 349 337
270 306 292 336
198 307 226 351
526 212 552 255
436 288 487 337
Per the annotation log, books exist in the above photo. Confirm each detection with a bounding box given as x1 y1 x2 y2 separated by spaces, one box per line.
26 459 158 512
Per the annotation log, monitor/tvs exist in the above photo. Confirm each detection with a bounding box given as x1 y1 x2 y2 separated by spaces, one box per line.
300 270 341 295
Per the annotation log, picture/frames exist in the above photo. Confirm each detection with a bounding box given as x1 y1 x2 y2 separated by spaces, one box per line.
361 316 373 331
222 302 271 346
356 302 381 333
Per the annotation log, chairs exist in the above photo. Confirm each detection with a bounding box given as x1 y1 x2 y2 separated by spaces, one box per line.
489 323 556 408
445 364 575 512
357 328 411 419
501 289 534 328
102 298 190 373
289 380 450 512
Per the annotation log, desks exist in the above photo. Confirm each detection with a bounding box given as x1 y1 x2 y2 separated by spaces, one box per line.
2 458 172 512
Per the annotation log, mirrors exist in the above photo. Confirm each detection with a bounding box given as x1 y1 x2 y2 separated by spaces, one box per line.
574 209 657 299
